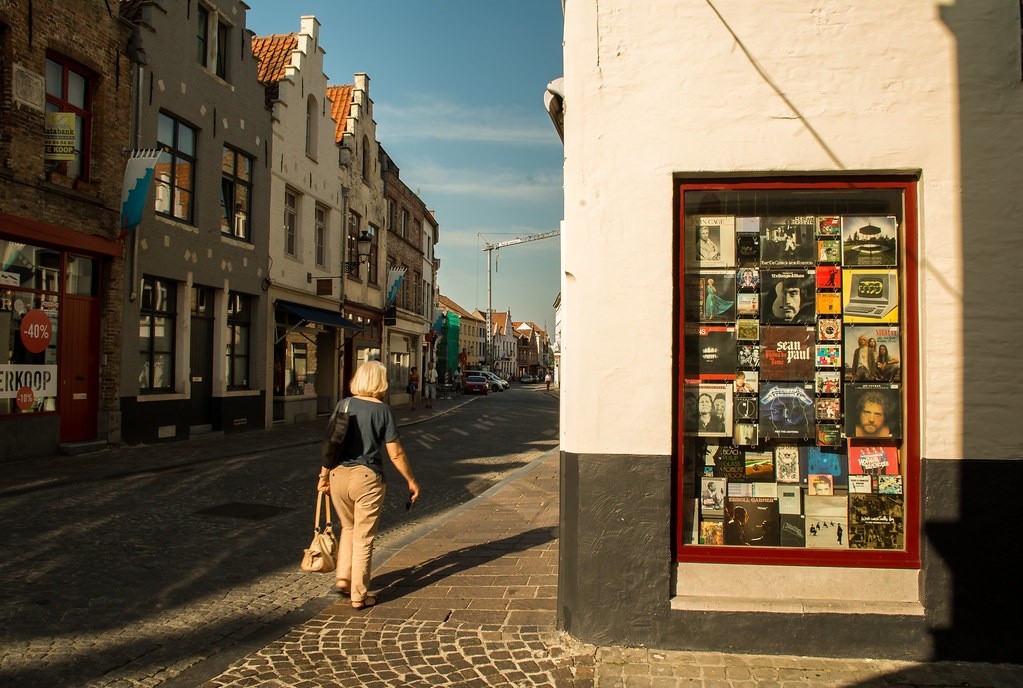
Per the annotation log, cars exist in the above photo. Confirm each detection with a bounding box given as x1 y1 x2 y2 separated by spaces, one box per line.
520 374 540 384
463 375 490 395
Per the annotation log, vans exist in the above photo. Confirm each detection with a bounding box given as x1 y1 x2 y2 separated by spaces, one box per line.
461 370 510 392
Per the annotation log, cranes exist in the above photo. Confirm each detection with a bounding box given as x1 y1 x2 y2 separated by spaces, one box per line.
477 229 560 373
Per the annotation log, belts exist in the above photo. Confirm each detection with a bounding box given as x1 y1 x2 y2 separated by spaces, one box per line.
426 381 436 384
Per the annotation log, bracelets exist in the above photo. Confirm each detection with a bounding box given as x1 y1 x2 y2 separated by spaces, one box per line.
319 474 329 477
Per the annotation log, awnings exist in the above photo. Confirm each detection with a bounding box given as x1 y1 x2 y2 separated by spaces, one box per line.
532 364 545 369
274 298 365 349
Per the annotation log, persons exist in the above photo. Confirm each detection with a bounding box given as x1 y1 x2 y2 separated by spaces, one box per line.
703 480 778 545
445 366 464 395
849 391 898 437
743 436 901 549
699 326 758 432
317 361 421 611
409 367 419 410
816 354 841 443
851 336 900 382
424 362 438 409
696 218 838 324
545 373 551 392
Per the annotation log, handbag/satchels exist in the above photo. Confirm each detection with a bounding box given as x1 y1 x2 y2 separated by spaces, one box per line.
318 397 352 469
406 383 416 394
301 491 339 574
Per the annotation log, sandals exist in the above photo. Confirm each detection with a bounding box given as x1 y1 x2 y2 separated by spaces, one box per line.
333 577 351 598
351 599 380 610
